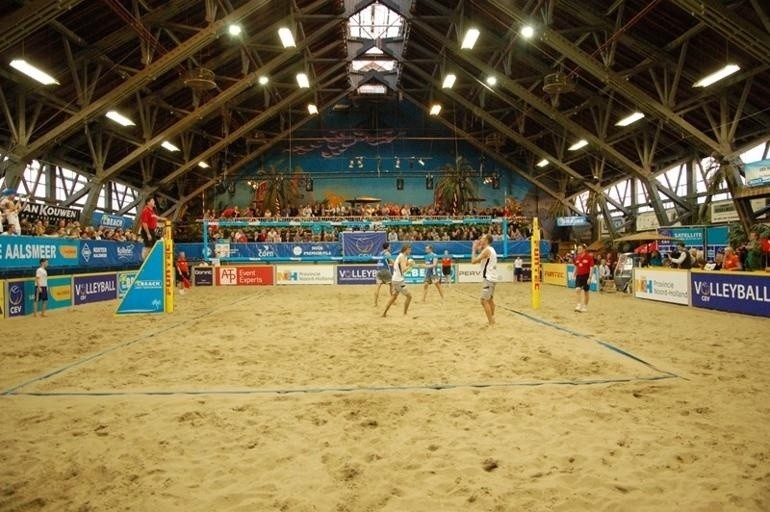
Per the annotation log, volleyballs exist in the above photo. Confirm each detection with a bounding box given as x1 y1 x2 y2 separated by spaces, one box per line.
408 258 415 266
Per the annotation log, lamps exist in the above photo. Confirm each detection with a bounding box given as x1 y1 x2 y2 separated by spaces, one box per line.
226 177 502 192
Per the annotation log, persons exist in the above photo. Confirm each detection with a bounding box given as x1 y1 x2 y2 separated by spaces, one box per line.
418 244 444 302
176 251 190 295
441 250 453 288
667 229 764 272
561 242 631 313
373 243 396 307
381 243 415 317
33 258 48 317
513 257 523 281
0 193 142 242
471 235 497 329
139 198 167 259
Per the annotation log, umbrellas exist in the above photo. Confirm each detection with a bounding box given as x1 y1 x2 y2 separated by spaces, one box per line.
613 231 681 268
